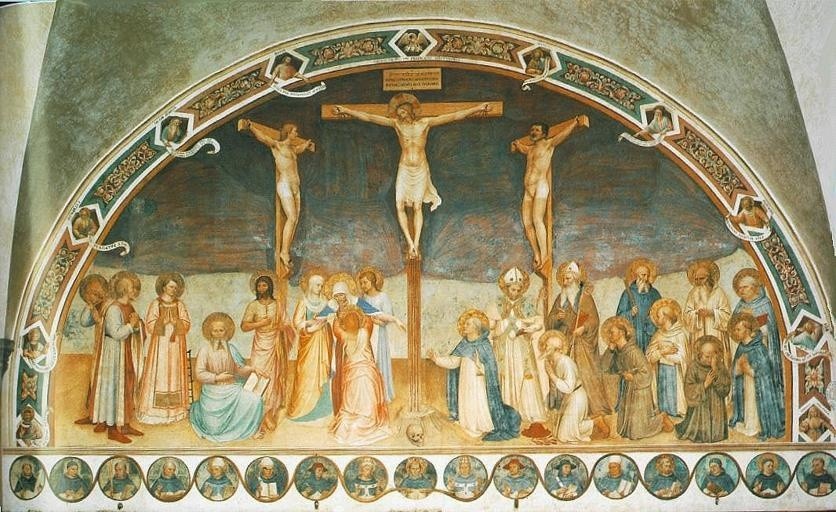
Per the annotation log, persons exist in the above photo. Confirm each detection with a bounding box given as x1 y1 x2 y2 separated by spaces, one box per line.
16 406 39 446
21 327 45 358
799 407 836 442
77 252 789 441
524 47 545 78
161 117 185 146
330 89 493 256
506 114 588 269
631 107 672 136
71 206 96 242
239 111 316 271
728 196 764 226
268 54 308 88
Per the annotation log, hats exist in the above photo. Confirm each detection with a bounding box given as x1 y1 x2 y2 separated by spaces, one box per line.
257 457 274 470
608 455 622 466
521 422 551 438
553 459 577 470
307 462 328 472
502 459 526 471
